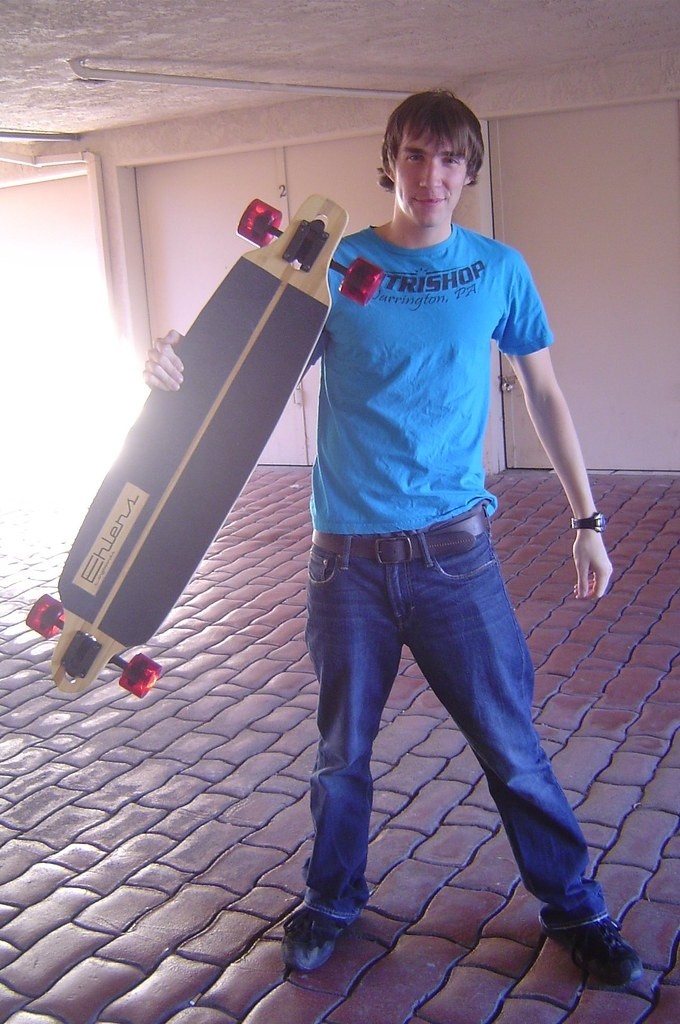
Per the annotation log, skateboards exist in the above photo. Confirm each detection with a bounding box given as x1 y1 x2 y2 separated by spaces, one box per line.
24 196 385 699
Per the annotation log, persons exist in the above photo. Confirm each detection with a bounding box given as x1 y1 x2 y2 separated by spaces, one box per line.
143 92 642 982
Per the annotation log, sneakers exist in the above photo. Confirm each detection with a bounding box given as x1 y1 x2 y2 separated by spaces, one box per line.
280 905 345 972
538 910 645 992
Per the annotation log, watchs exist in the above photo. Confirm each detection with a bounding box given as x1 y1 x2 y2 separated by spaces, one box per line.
571 512 607 532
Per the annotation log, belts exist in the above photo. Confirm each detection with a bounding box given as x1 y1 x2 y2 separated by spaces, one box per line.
311 499 491 565
